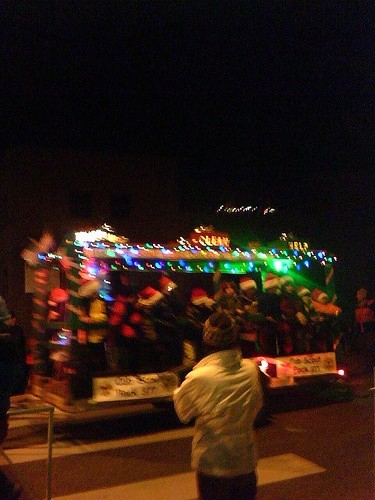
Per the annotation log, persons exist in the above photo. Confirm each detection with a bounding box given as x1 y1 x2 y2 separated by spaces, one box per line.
96 268 375 386
172 305 263 500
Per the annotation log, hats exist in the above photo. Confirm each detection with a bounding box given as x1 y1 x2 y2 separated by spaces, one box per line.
239 277 258 291
279 275 294 285
311 288 329 303
264 273 281 289
203 309 239 351
159 276 177 296
297 286 310 296
79 278 100 297
138 286 165 305
191 288 208 305
